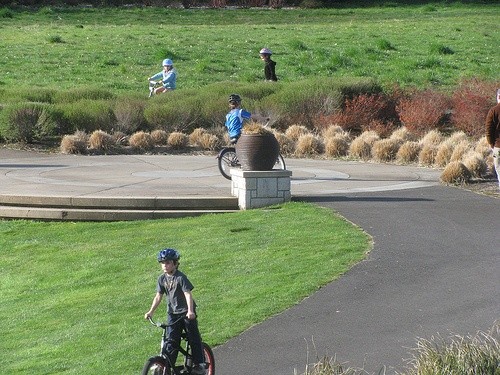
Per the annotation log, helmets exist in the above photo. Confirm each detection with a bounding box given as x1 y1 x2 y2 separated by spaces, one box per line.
162 58 174 67
157 248 181 263
260 48 272 55
229 94 242 104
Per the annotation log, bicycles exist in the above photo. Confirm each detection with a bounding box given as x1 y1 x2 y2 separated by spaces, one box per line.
147 80 159 98
140 316 215 375
214 117 286 180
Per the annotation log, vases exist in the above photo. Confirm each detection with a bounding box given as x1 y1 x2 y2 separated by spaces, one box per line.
235 131 280 171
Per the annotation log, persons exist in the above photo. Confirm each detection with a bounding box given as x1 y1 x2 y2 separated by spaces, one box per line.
225 94 271 138
145 248 210 375
148 58 176 94
259 48 278 82
485 89 500 187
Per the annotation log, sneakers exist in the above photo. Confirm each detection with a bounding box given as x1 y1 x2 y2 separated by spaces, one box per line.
190 365 208 375
